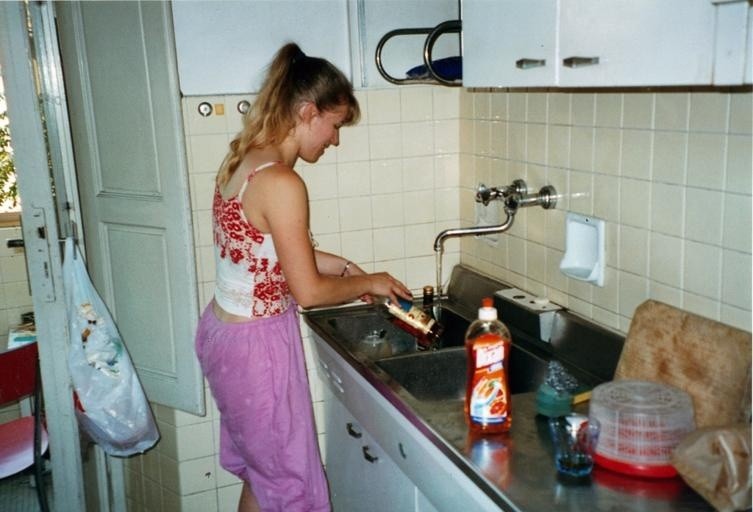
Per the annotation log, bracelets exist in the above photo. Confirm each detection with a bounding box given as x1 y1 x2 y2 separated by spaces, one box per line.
340 259 352 278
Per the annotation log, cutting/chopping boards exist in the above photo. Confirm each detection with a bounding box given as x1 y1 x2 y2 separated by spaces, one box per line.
613 298 751 433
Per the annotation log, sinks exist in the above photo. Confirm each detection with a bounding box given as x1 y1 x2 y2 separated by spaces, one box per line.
375 342 547 401
325 304 471 357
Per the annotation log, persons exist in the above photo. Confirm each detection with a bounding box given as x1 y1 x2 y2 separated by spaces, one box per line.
194 41 415 512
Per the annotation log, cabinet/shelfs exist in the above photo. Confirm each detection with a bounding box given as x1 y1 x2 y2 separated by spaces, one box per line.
173 2 461 98
460 1 753 91
308 385 416 512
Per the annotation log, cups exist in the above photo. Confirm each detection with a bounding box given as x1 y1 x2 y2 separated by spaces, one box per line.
548 410 602 479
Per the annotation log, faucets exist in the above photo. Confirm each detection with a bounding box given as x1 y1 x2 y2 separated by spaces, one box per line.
433 198 516 252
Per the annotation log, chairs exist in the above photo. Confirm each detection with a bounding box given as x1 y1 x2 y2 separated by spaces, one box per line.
0 341 46 511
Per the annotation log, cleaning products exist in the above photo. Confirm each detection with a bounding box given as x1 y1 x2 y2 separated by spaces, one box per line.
536 360 579 418
461 436 512 493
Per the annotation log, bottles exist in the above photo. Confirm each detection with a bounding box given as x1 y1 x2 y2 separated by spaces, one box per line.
361 291 443 349
